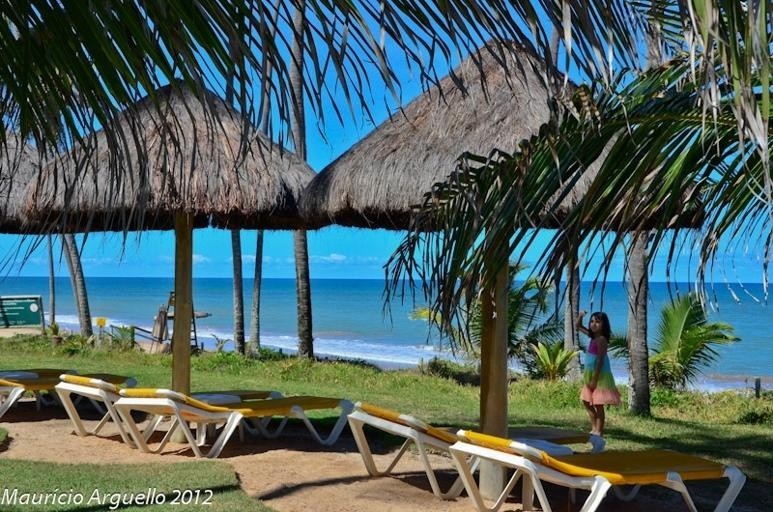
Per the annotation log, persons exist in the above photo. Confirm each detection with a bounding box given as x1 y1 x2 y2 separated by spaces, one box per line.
573 309 623 438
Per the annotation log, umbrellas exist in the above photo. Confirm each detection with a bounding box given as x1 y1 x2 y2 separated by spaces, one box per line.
0 125 62 237
19 77 334 446
297 35 706 501
